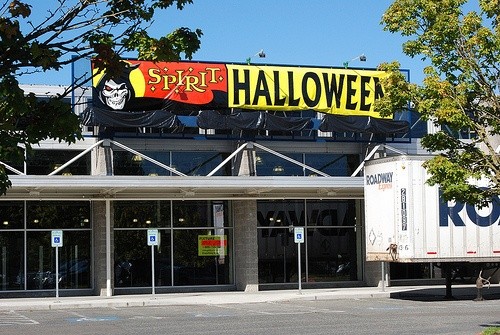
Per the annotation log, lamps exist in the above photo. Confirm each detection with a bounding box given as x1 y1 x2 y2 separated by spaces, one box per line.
342 53 367 69
246 49 266 65
